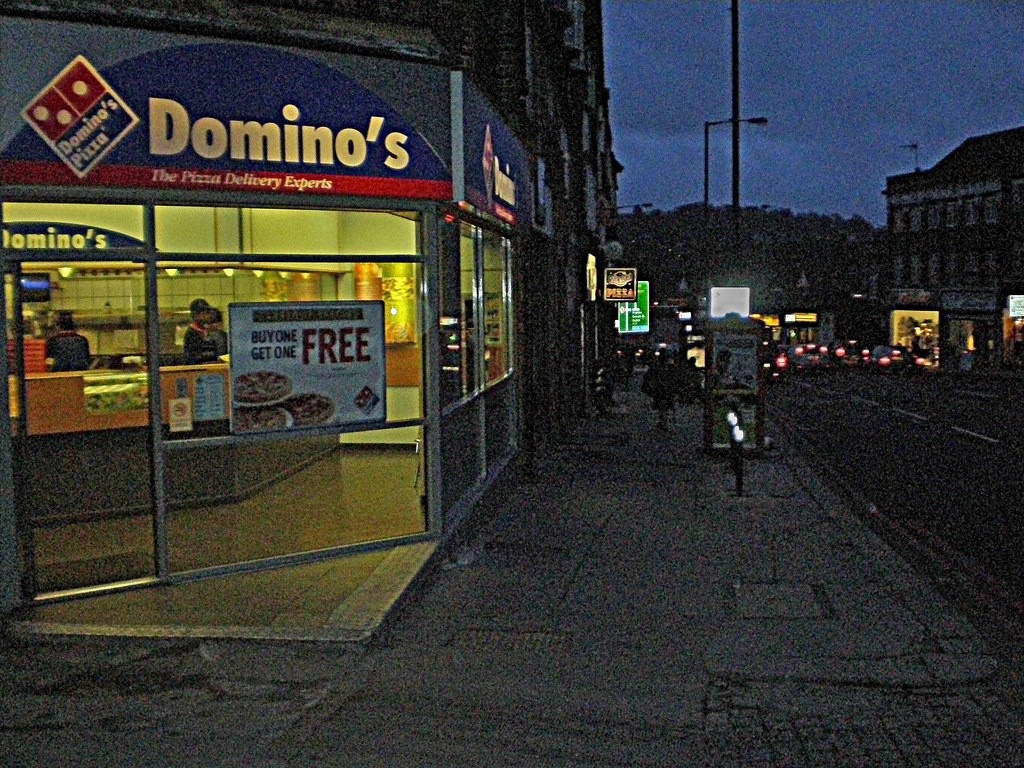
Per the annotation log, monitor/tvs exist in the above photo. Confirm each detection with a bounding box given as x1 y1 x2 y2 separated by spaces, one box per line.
20 273 52 302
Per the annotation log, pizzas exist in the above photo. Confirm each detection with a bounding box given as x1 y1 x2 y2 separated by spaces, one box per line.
232 370 334 431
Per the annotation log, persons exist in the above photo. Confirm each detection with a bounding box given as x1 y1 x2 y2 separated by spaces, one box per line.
44 313 89 372
207 308 228 355
183 299 216 365
118 315 132 330
641 353 681 429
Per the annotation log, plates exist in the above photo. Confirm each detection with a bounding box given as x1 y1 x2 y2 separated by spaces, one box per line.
232 369 294 406
276 407 293 429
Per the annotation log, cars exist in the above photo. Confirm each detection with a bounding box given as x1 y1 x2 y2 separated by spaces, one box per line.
642 335 913 438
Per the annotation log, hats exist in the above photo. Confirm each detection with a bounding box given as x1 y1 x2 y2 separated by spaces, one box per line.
52 312 73 324
189 299 213 312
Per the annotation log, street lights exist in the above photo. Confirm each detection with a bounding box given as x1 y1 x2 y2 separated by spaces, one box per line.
701 114 769 283
